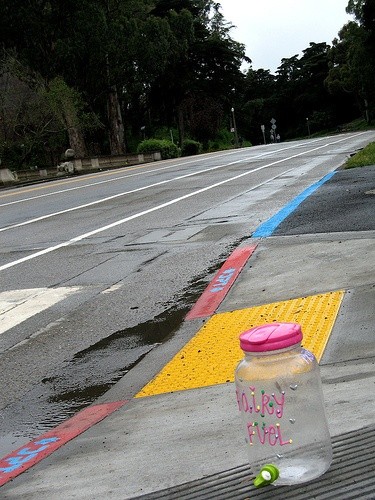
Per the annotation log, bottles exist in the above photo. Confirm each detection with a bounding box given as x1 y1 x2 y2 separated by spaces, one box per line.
235 321 334 488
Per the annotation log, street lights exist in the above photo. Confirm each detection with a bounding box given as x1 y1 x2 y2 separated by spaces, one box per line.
307 117 312 138
231 108 240 148
